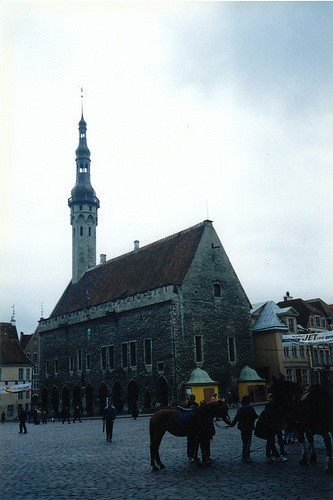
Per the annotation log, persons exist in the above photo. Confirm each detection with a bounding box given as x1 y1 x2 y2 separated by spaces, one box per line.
31 404 96 424
231 394 260 462
130 400 139 420
151 398 156 408
103 401 117 442
183 391 234 420
17 408 27 434
1 411 6 424
259 394 298 462
184 394 216 464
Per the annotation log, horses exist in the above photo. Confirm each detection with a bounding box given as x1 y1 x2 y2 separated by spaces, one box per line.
271 374 333 472
149 396 231 471
